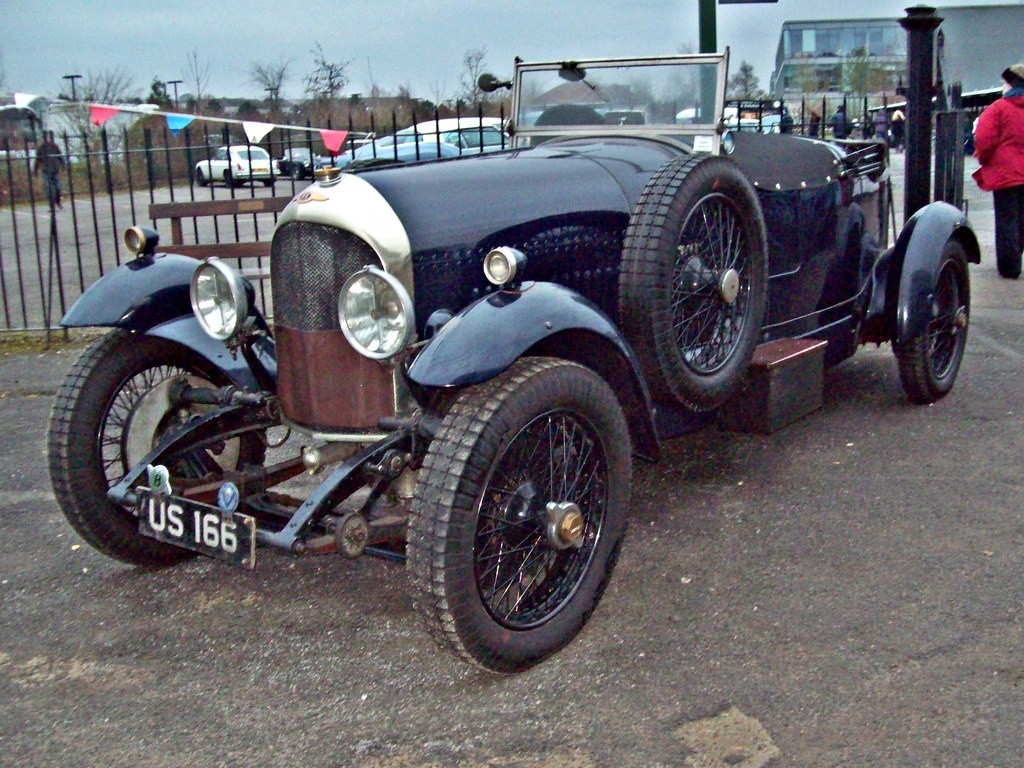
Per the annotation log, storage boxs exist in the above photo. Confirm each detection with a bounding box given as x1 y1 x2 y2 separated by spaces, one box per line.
717 337 830 435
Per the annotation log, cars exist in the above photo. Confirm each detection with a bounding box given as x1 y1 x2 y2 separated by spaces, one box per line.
277 107 760 180
47 47 982 677
195 146 282 189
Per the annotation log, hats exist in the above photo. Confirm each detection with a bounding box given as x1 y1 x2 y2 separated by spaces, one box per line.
1001 64 1024 87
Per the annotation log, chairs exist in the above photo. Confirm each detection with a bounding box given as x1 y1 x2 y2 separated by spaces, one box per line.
725 131 845 258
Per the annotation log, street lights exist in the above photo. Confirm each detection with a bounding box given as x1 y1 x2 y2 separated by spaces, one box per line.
265 88 278 100
63 75 83 101
167 81 183 109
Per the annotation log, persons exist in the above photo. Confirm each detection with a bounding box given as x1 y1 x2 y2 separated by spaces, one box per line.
32 131 67 211
779 108 794 135
825 105 853 140
863 108 905 154
972 64 1024 279
805 111 822 137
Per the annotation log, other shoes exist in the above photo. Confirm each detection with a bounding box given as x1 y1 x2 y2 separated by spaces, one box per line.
56 200 62 209
